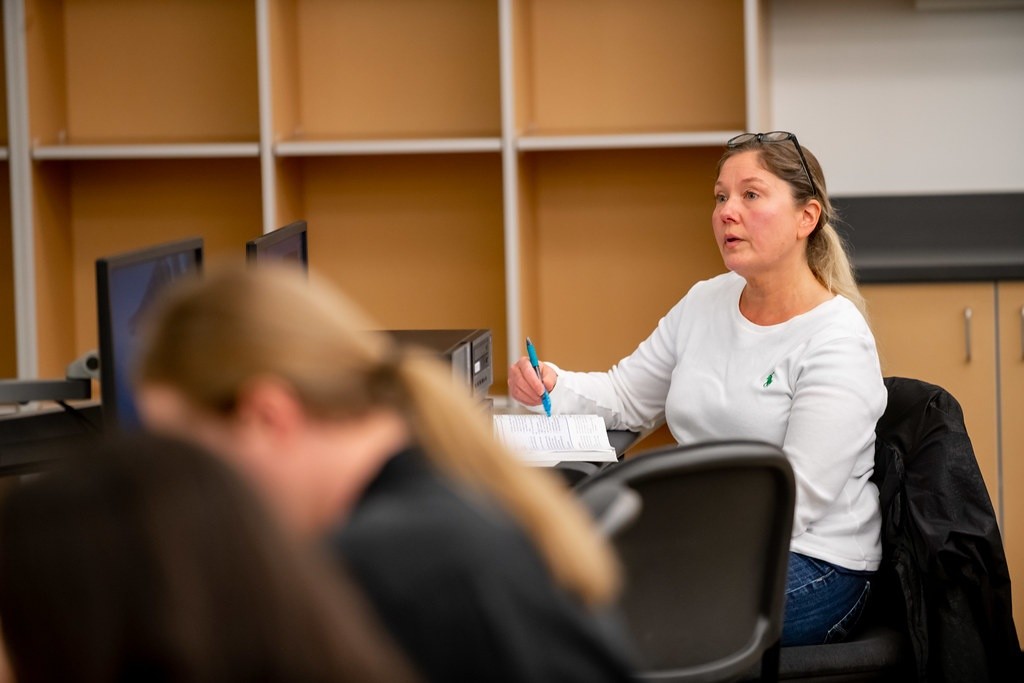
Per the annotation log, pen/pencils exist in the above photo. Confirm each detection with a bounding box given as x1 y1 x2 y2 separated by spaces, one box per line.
526 335 551 417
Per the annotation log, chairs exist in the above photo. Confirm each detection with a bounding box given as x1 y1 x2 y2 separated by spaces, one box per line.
571 436 800 683
778 376 969 683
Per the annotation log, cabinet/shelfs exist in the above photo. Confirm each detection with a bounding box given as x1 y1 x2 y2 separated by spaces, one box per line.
829 191 1024 650
0 0 776 398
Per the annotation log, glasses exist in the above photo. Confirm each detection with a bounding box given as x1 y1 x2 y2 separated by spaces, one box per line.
726 131 817 198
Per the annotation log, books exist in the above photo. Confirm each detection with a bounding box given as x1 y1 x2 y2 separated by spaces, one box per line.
491 413 622 468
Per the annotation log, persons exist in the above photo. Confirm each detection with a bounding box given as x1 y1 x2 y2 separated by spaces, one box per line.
1 264 648 683
507 134 887 648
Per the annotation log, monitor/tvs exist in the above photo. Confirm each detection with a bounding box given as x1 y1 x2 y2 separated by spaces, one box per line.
96 237 204 440
245 219 308 275
